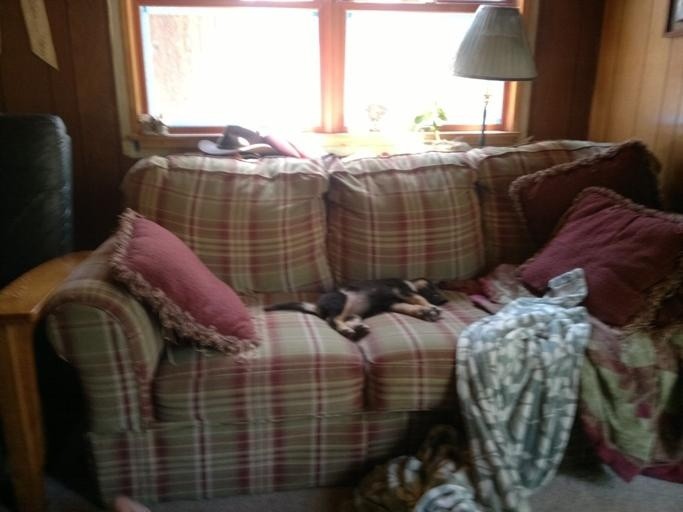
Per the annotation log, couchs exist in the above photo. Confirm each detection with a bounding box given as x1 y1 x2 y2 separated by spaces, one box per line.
38 135 682 511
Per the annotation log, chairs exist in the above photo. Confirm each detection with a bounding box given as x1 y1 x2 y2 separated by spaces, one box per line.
1 114 92 512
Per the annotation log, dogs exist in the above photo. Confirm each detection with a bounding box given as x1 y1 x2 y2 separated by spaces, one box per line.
262 277 450 341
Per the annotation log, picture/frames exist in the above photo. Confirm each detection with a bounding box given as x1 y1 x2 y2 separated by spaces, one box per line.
661 0 683 39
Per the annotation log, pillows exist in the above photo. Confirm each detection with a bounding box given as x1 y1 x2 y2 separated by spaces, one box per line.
106 202 265 362
513 181 683 339
508 137 668 256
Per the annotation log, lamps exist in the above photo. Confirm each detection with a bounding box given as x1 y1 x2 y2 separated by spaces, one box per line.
450 4 542 153
